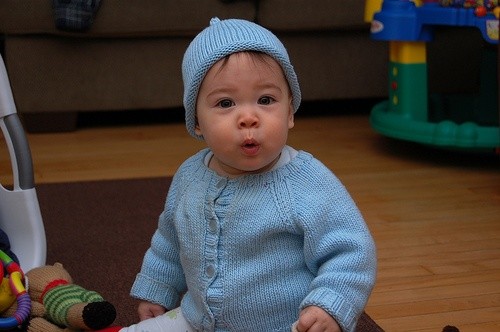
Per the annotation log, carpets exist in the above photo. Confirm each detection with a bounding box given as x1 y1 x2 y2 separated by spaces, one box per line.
3 176 385 331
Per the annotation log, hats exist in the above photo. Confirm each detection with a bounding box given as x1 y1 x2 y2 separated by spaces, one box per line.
182 16 301 140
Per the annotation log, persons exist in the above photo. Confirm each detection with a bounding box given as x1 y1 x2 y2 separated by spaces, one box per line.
95 16 460 332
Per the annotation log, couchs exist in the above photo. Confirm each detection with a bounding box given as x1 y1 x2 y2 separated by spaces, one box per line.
0 1 389 134
